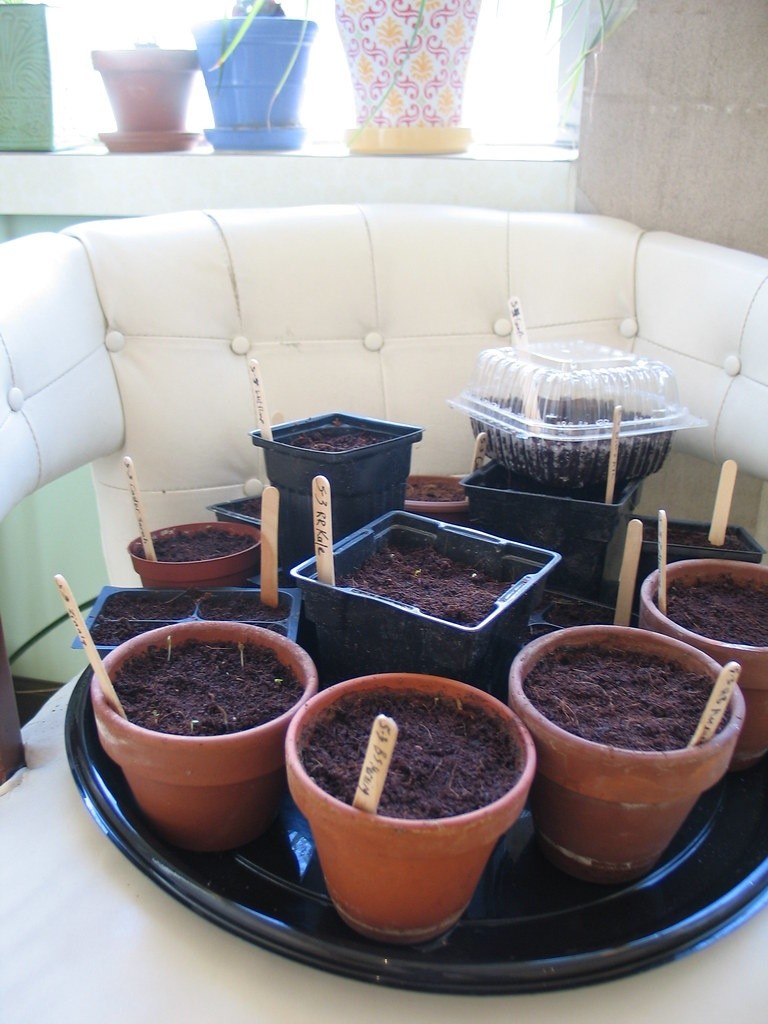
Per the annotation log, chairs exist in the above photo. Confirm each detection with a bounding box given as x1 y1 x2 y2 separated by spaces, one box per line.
0 202 768 799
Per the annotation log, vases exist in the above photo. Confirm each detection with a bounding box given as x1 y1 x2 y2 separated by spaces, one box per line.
93 621 318 853
286 673 537 945
459 456 643 612
248 412 425 540
206 495 262 526
640 561 768 773
128 522 261 589
290 512 562 703
91 1 482 154
628 513 768 564
509 625 745 886
